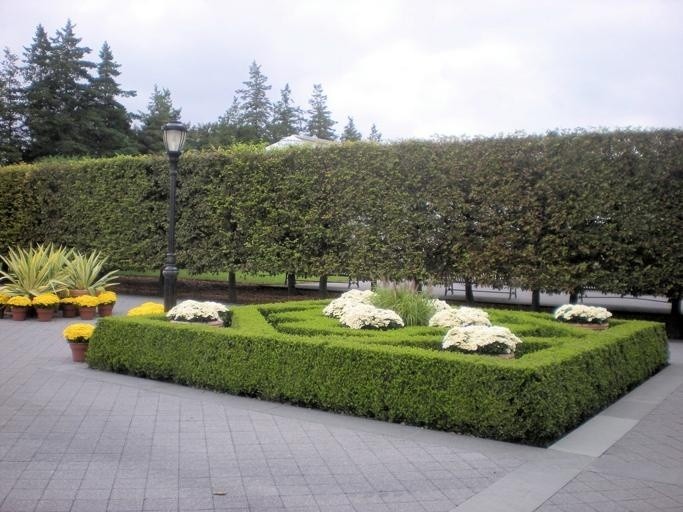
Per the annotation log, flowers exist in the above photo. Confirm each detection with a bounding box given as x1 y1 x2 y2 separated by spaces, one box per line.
441 324 523 354
166 299 220 322
554 304 612 324
0 289 165 342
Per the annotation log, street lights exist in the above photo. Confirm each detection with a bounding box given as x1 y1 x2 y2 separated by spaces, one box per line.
160 116 187 312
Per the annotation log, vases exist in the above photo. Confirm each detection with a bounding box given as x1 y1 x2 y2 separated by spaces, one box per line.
498 353 515 359
170 319 224 329
69 343 88 361
570 323 609 331
